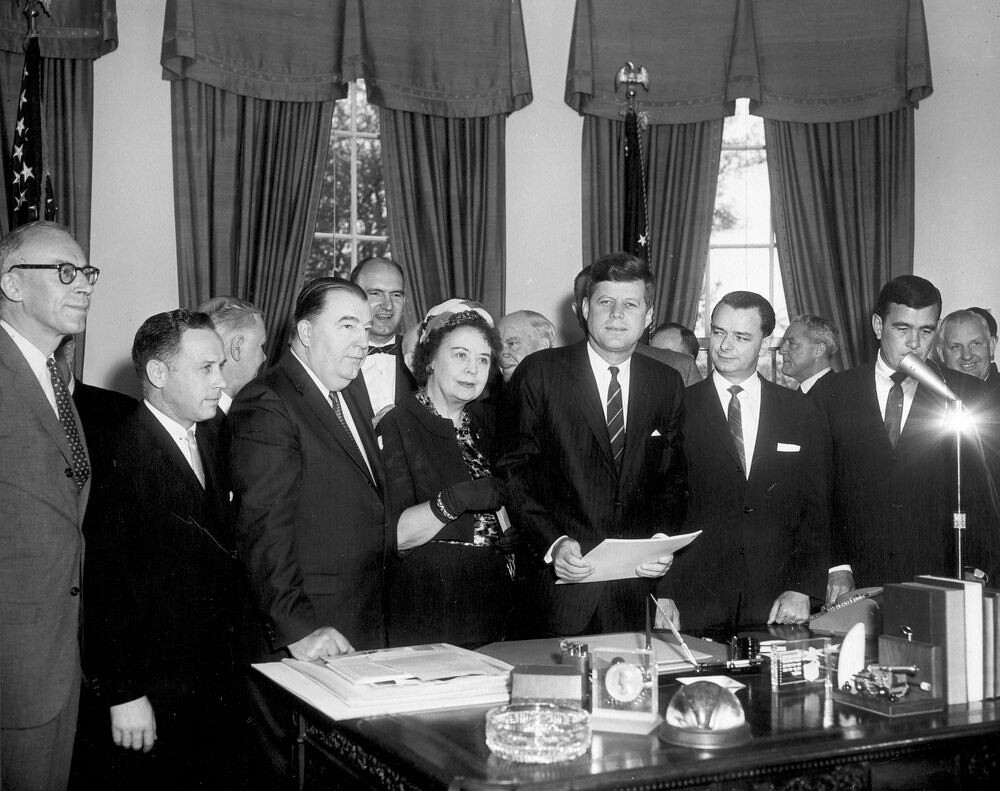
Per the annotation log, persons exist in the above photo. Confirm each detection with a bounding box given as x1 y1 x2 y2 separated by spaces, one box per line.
342 255 419 427
662 294 835 629
571 265 705 390
221 278 394 791
377 298 529 652
779 313 845 396
195 296 269 447
647 322 701 366
51 333 140 493
83 308 252 791
498 253 692 639
496 310 555 383
0 220 95 791
937 309 1000 392
824 276 1000 614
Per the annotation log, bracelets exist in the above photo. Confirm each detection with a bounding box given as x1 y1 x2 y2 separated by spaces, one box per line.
435 489 459 522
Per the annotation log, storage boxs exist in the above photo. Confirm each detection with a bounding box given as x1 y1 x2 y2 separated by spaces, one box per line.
510 664 584 709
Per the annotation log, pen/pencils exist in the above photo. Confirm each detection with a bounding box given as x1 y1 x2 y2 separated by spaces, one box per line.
649 593 698 668
644 595 652 676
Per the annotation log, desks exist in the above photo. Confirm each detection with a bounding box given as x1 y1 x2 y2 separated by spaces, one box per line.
288 622 999 791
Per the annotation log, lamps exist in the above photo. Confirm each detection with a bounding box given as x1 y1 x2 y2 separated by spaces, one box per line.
898 353 968 580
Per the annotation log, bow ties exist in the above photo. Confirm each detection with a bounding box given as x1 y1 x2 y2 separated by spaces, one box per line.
367 344 398 356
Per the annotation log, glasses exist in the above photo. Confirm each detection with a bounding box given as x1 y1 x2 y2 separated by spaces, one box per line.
8 262 100 286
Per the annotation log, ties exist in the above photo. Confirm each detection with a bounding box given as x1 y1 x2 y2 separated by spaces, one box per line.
46 358 90 494
329 390 370 474
883 372 909 450
607 367 625 476
726 385 746 481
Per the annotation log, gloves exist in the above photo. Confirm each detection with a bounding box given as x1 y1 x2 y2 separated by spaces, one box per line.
429 476 510 524
494 525 522 555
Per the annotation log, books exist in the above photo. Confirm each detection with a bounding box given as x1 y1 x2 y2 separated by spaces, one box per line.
881 574 1000 705
